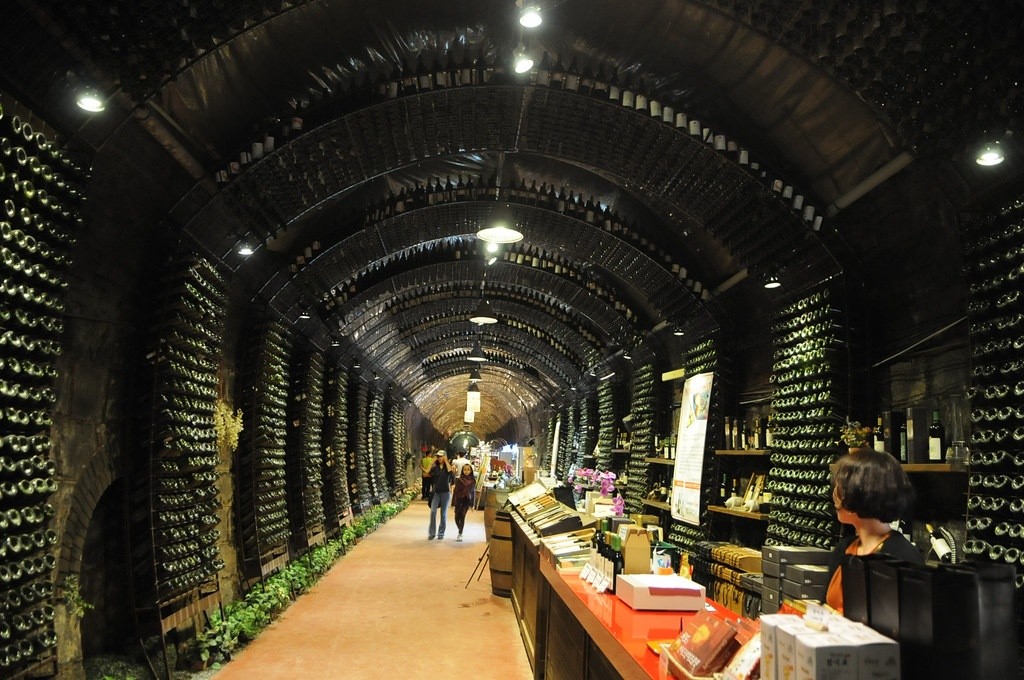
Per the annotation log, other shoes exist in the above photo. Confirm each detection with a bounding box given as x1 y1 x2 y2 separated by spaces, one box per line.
425 497 429 501
438 535 444 539
457 534 463 542
421 496 426 500
428 535 435 540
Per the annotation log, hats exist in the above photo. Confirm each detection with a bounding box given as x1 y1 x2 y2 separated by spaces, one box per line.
433 449 447 456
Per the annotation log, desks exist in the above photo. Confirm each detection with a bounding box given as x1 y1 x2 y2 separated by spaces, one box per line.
539 552 762 680
510 511 541 680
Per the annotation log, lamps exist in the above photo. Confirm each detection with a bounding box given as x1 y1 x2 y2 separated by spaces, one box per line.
673 324 684 336
590 347 634 377
293 302 311 319
761 263 781 289
976 128 1015 166
326 334 340 347
516 0 543 28
351 360 379 380
468 192 523 392
67 71 106 112
514 41 534 74
225 228 254 256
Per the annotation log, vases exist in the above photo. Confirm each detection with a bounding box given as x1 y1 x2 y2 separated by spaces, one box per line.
849 448 861 455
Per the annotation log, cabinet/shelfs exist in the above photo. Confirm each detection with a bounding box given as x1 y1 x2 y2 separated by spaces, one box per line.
898 463 968 569
583 449 770 521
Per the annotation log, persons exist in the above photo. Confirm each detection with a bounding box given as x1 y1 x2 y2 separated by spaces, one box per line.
419 451 437 498
820 451 921 613
448 449 472 507
423 450 455 539
452 463 476 541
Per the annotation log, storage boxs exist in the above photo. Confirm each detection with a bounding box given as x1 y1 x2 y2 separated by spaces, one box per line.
617 524 650 574
616 573 706 610
758 546 1017 680
906 406 929 464
516 496 596 576
882 411 905 462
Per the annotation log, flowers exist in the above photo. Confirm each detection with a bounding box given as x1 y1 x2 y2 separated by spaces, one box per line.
834 416 873 448
568 464 626 518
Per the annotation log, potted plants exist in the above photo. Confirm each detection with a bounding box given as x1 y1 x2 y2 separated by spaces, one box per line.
179 627 224 671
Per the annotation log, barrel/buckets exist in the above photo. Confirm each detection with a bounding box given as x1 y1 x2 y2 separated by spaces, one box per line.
489 510 513 598
484 493 498 542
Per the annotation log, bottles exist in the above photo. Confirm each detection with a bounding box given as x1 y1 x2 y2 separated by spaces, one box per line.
591 518 624 595
663 436 676 459
928 411 945 463
952 440 963 457
750 475 770 514
724 416 775 450
925 522 952 563
731 478 737 498
874 418 885 453
721 473 727 507
615 428 623 448
